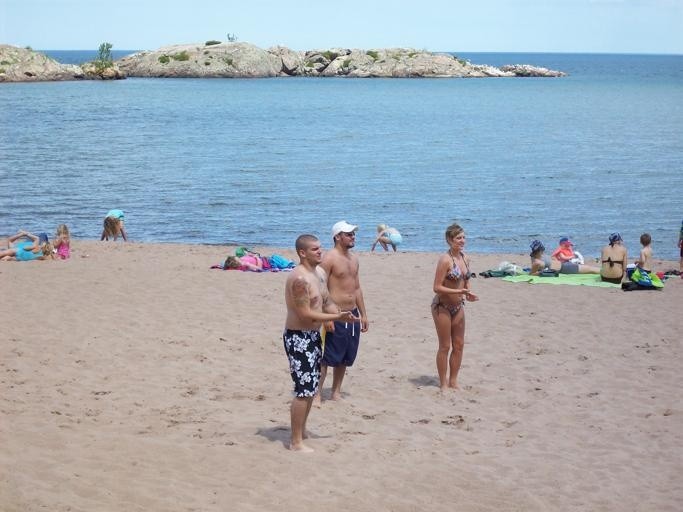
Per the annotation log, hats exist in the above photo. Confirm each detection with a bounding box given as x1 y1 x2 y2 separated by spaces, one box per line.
609 232 623 241
330 220 360 237
528 239 545 256
560 238 574 249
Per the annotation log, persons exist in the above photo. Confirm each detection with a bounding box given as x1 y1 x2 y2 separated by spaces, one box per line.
223 253 296 272
529 239 600 276
0 224 70 261
311 221 370 408
101 209 126 241
634 233 654 274
600 232 627 284
678 220 683 273
371 224 403 252
552 237 580 265
282 234 360 451
430 223 479 391
